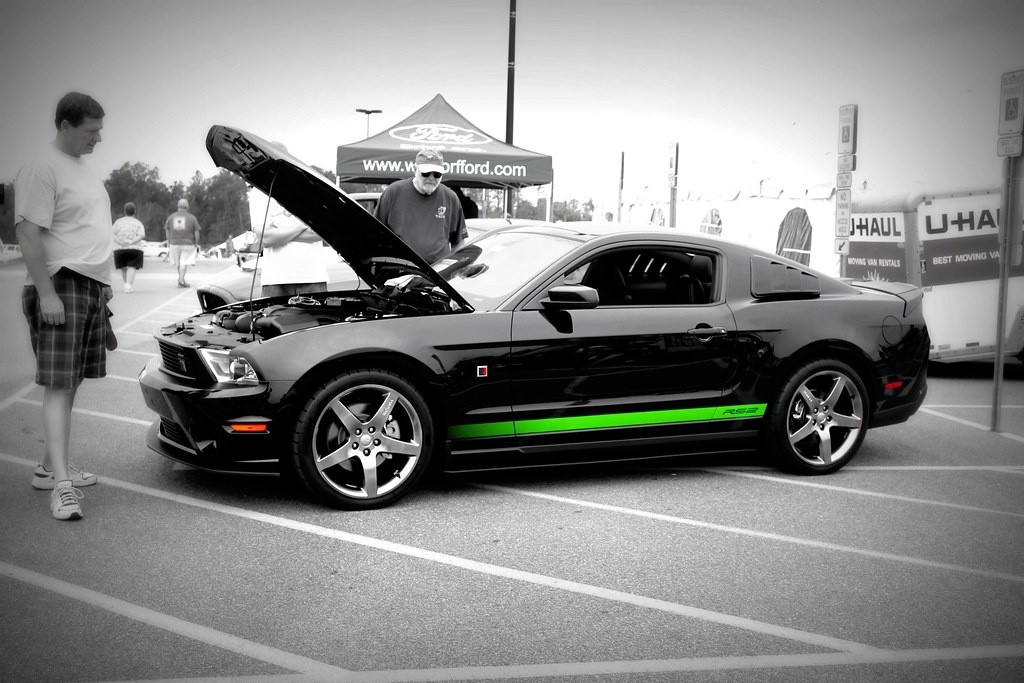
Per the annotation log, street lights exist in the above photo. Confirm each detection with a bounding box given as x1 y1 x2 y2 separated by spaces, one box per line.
356 109 382 138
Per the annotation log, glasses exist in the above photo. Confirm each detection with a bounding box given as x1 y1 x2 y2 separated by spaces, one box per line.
421 172 442 178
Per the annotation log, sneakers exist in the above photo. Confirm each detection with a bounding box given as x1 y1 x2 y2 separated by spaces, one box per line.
32 462 96 490
50 480 84 520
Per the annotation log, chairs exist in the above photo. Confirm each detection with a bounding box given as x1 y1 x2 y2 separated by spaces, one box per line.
664 277 706 304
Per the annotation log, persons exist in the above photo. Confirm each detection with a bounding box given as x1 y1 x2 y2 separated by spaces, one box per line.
374 149 469 265
451 187 478 219
14 92 112 519
112 203 145 293
226 235 234 257
252 202 328 296
165 199 201 288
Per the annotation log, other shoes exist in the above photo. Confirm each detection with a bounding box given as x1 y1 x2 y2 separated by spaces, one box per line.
123 285 135 292
179 281 190 287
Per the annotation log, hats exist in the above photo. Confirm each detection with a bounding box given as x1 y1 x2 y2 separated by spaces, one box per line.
178 199 188 207
415 149 445 175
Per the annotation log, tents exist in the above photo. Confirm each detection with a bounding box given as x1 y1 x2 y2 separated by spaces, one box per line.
336 94 553 222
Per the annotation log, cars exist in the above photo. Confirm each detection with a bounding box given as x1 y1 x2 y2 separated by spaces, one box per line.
138 126 931 509
142 240 168 257
1 245 20 254
